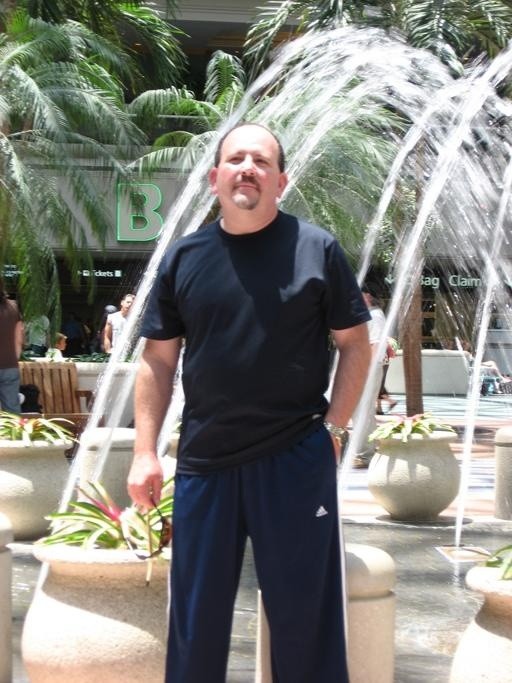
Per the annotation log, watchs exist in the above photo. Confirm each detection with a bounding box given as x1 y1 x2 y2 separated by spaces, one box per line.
324 420 349 447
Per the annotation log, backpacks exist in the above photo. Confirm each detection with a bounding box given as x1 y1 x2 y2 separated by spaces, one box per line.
387 337 400 357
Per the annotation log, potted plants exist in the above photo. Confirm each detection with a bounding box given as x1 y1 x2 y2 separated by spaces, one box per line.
448 545 512 683
20 473 175 683
0 410 80 541
367 412 462 521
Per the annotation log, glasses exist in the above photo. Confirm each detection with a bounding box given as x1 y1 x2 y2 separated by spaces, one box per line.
122 497 173 559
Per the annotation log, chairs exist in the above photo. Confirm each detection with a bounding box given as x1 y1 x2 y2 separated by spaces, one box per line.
17 360 106 458
453 336 501 396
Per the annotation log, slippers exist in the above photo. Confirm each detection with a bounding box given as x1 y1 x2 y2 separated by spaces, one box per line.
376 401 397 414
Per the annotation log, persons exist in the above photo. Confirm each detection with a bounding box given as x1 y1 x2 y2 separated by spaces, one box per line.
351 284 385 468
376 350 397 415
100 312 112 350
462 340 510 393
45 332 68 359
24 310 51 357
2 278 24 413
125 124 371 682
104 293 136 353
